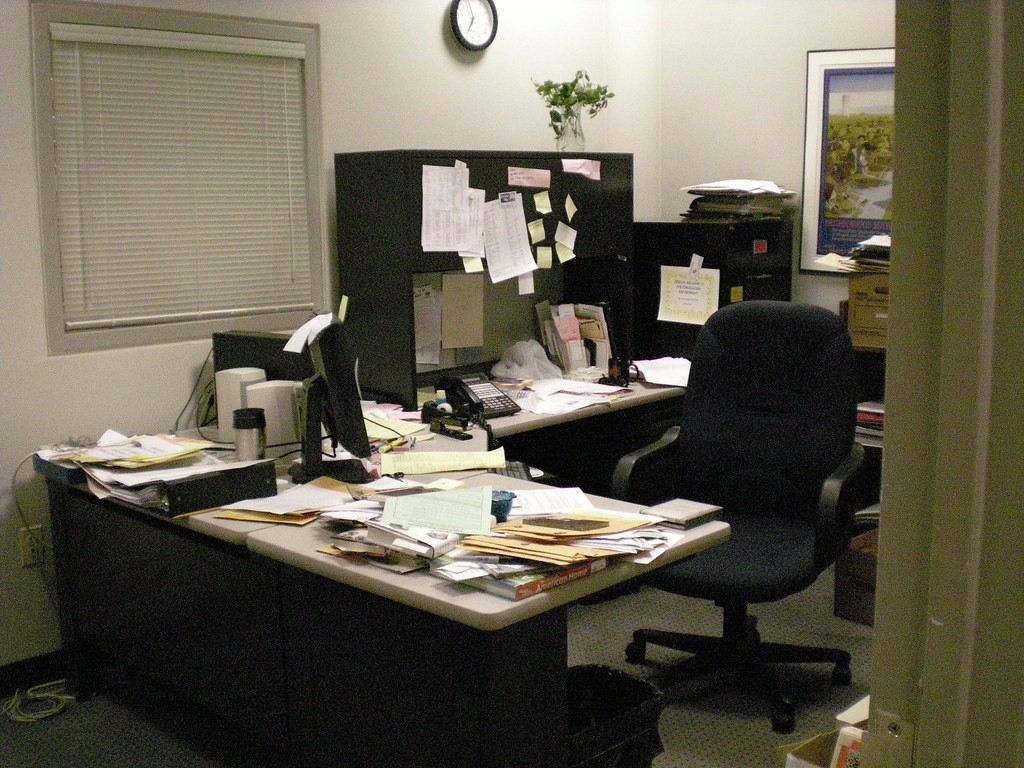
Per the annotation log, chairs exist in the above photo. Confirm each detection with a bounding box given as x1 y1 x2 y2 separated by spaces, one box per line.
610 300 865 735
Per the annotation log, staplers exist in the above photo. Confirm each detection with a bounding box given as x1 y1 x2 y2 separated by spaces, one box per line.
430 416 473 441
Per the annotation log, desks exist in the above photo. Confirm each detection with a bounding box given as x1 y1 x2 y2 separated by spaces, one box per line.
245 473 732 768
32 358 685 768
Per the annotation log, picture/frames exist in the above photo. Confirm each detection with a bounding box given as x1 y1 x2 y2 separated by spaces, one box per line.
797 46 895 276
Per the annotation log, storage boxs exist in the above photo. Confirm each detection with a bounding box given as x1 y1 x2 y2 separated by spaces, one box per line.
848 272 889 348
785 695 871 768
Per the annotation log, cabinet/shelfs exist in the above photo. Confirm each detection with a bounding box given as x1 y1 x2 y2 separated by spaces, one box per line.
332 147 636 411
832 300 887 630
633 218 795 363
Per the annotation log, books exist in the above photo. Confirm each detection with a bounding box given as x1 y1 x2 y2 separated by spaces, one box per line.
856 401 884 439
679 179 798 222
331 513 607 602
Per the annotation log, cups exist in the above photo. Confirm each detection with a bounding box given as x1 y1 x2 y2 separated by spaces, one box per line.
608 358 638 383
233 409 267 461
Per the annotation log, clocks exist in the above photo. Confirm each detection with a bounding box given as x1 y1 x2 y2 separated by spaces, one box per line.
449 0 498 52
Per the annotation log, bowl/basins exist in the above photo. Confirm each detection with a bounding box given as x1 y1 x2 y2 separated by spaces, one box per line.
491 492 516 521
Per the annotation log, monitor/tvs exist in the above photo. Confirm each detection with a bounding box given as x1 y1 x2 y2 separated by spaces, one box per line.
289 322 372 486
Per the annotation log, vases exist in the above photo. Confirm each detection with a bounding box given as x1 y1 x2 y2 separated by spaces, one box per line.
557 102 586 152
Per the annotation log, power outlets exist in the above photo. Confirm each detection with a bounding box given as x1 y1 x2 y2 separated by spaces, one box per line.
18 525 44 568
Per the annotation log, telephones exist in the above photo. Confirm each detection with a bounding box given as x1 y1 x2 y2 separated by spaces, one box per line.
446 372 521 421
